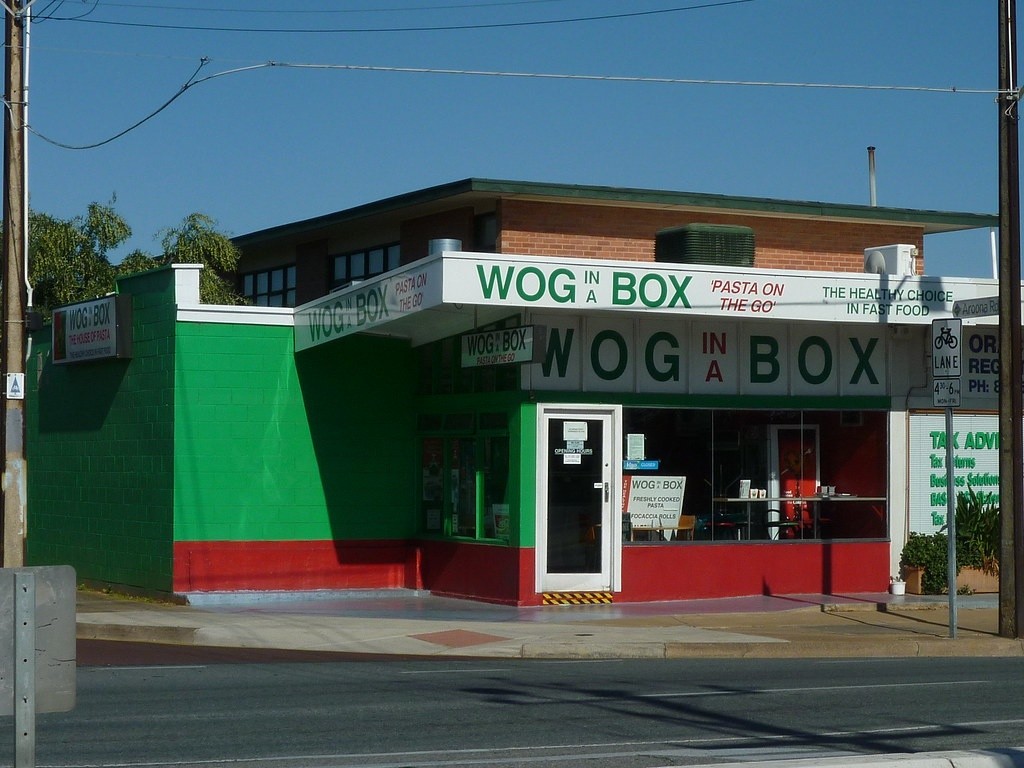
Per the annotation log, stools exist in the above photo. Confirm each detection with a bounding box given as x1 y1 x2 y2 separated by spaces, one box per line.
762 510 799 540
727 513 755 540
578 513 601 571
798 509 832 539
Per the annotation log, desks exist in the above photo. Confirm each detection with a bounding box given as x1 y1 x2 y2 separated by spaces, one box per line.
713 497 886 541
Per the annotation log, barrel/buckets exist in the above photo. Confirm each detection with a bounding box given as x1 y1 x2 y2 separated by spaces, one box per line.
890 582 906 595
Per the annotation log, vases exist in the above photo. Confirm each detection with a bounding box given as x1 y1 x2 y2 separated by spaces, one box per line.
890 581 907 595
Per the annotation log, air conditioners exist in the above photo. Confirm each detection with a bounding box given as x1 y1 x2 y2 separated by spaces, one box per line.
864 244 917 275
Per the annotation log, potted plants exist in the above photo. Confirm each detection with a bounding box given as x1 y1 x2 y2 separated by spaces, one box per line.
899 482 1000 595
